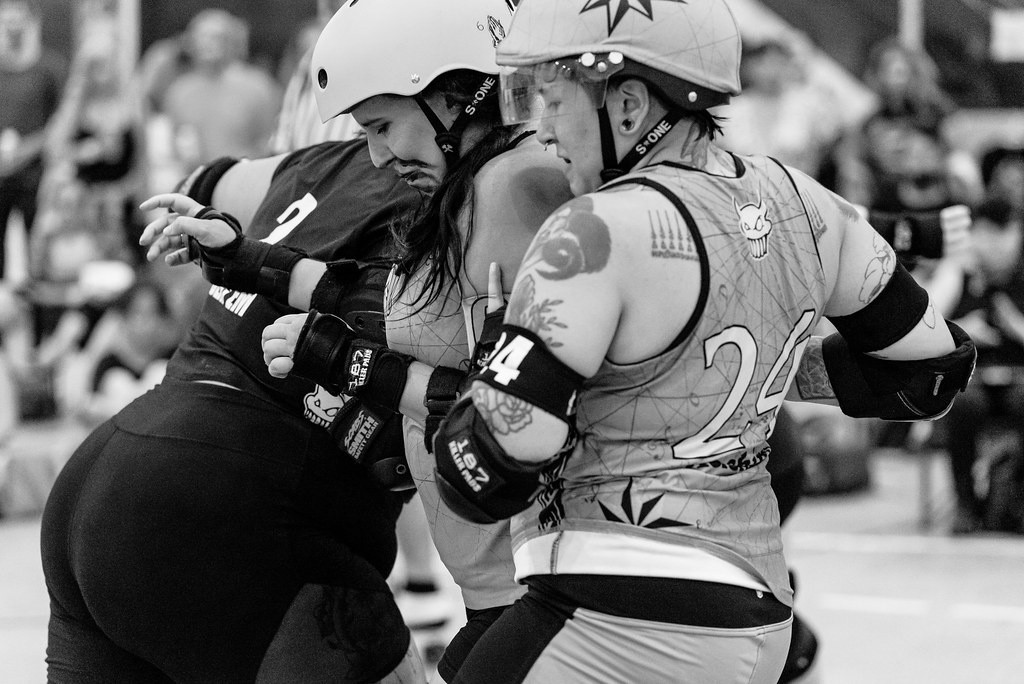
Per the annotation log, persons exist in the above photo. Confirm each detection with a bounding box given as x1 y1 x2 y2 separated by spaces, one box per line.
848 42 1024 536
433 2 970 684
3 0 368 450
392 484 447 625
139 0 576 684
40 144 442 682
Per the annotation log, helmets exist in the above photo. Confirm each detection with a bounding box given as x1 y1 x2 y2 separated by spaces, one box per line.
309 1 502 207
492 0 742 128
